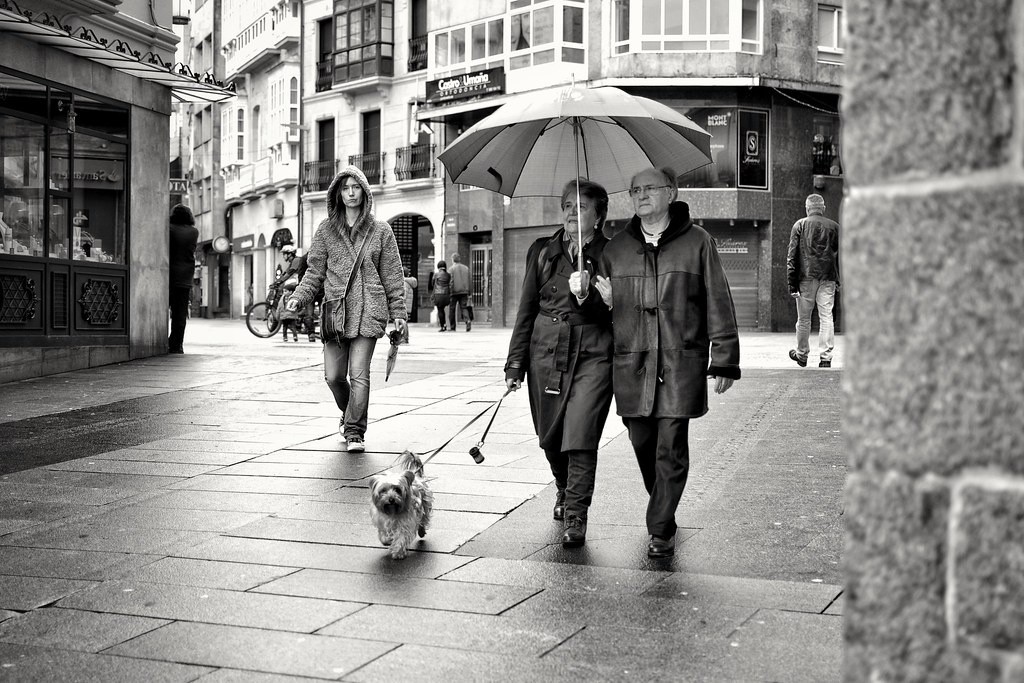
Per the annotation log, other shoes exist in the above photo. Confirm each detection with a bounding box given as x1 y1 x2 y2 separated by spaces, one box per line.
438 327 447 332
339 414 345 434
309 336 315 341
819 361 831 367
466 321 471 331
347 437 365 451
789 350 806 366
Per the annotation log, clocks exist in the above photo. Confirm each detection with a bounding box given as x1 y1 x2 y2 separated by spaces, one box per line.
212 235 230 254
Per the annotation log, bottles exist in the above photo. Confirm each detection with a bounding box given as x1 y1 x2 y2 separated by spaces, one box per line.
33 235 43 257
80 251 86 260
96 252 113 262
5 227 12 252
58 246 67 257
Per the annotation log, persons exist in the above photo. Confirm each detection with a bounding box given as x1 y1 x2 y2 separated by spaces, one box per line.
430 261 451 331
269 245 317 341
568 168 740 560
787 194 840 367
285 165 407 452
504 177 613 545
402 267 417 343
168 205 198 353
448 252 472 331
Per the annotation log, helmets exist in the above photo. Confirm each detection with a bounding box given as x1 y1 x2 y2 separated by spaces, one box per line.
280 246 296 254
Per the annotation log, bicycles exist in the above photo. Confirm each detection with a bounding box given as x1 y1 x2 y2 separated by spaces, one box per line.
245 285 321 338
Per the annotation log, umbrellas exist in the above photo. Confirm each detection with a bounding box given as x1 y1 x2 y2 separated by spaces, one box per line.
435 73 716 304
385 327 404 382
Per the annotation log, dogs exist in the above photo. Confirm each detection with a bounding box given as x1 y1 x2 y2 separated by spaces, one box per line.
368 450 435 559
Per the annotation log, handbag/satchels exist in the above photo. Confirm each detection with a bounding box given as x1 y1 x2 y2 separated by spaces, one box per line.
320 298 346 342
461 298 473 320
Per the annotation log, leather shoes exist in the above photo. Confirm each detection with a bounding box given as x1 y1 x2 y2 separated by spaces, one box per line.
563 516 586 546
648 536 675 558
553 492 565 519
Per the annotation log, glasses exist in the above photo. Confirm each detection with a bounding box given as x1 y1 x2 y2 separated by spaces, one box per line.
629 185 672 198
